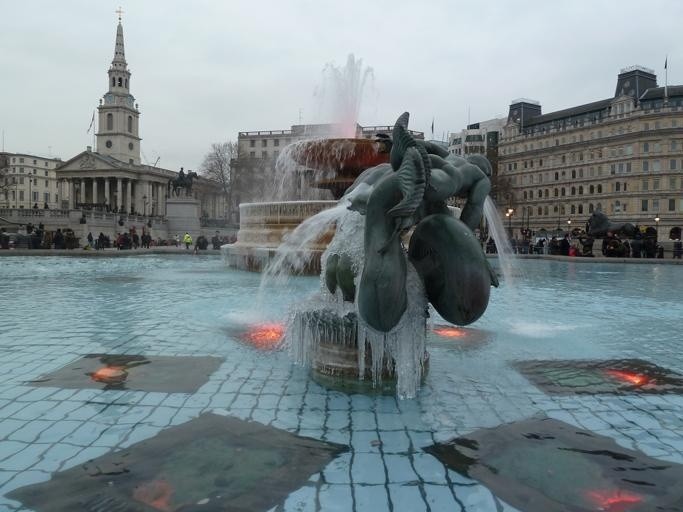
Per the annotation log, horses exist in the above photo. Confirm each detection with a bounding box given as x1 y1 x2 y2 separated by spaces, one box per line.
167 171 199 197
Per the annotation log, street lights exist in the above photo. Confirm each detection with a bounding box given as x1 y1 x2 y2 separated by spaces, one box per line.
505 207 516 235
566 217 572 235
653 212 660 231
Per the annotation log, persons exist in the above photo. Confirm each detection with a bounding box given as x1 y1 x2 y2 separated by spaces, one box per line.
18 201 237 250
179 167 187 184
519 226 683 258
